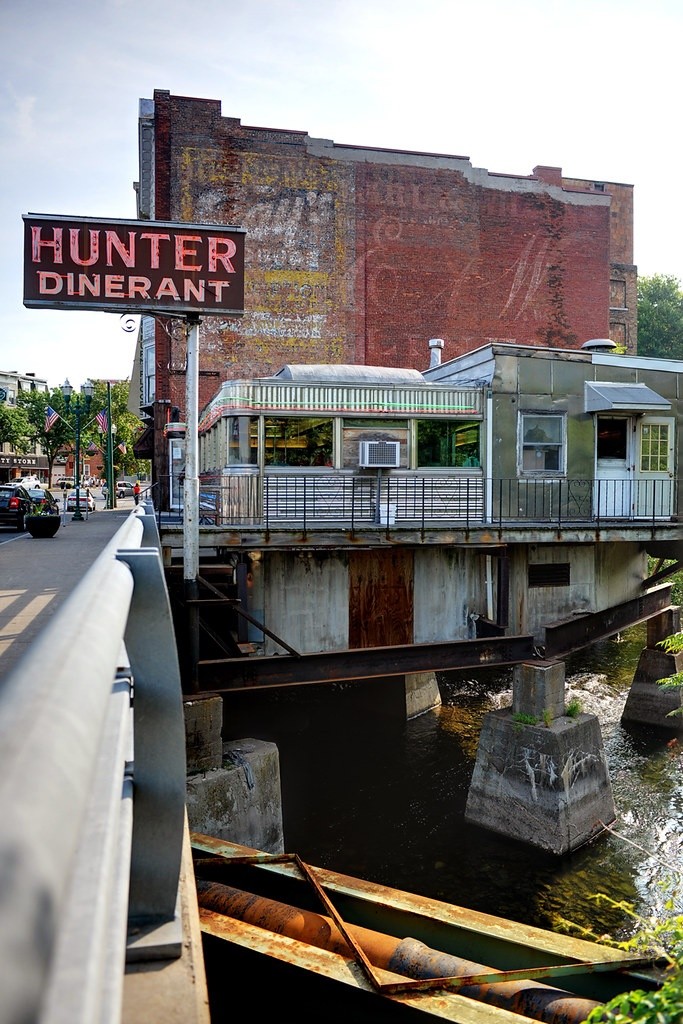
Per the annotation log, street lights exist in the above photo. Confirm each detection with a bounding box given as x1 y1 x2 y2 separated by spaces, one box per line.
61 377 96 520
98 424 117 507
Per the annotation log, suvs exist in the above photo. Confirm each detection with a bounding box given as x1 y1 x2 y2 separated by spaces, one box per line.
102 481 135 498
6 477 41 490
56 477 83 488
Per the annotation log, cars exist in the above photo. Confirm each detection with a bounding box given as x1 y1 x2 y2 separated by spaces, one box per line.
69 490 95 511
78 475 95 486
0 484 35 531
26 491 60 516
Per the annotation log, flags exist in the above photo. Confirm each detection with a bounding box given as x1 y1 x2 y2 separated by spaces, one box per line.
45 407 59 432
118 441 127 454
87 441 100 452
96 406 108 433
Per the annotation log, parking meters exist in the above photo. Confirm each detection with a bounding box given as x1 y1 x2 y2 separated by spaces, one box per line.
85 488 89 520
106 489 109 509
63 490 67 527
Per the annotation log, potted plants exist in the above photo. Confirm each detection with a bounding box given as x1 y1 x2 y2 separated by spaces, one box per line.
18 502 62 538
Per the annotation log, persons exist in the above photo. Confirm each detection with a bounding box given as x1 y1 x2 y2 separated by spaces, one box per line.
123 483 128 487
133 483 140 505
102 479 118 498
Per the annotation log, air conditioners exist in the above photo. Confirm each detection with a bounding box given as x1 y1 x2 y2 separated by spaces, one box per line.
359 440 400 467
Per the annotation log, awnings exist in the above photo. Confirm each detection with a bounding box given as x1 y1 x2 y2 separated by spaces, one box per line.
584 381 673 411
